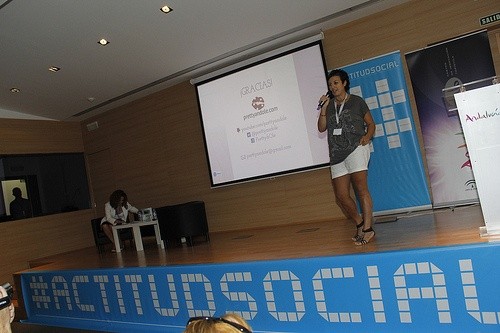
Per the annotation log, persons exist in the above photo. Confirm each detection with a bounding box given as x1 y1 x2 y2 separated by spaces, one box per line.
185 314 250 333
0 286 15 333
101 190 140 252
9 187 32 216
318 69 375 245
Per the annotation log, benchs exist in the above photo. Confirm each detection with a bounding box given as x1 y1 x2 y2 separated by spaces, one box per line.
90 201 210 251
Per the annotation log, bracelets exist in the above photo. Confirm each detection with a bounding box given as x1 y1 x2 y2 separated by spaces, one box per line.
320 113 327 116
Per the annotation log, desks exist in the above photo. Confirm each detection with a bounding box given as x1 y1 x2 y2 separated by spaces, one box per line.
112 220 163 252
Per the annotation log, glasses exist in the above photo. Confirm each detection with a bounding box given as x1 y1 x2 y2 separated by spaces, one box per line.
186 316 252 333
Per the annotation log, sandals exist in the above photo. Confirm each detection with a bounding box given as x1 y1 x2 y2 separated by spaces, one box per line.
354 226 375 246
352 220 364 242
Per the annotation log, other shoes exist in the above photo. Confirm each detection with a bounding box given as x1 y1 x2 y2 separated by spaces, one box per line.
112 246 123 252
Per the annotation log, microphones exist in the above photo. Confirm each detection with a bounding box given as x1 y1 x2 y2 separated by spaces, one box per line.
317 91 332 110
